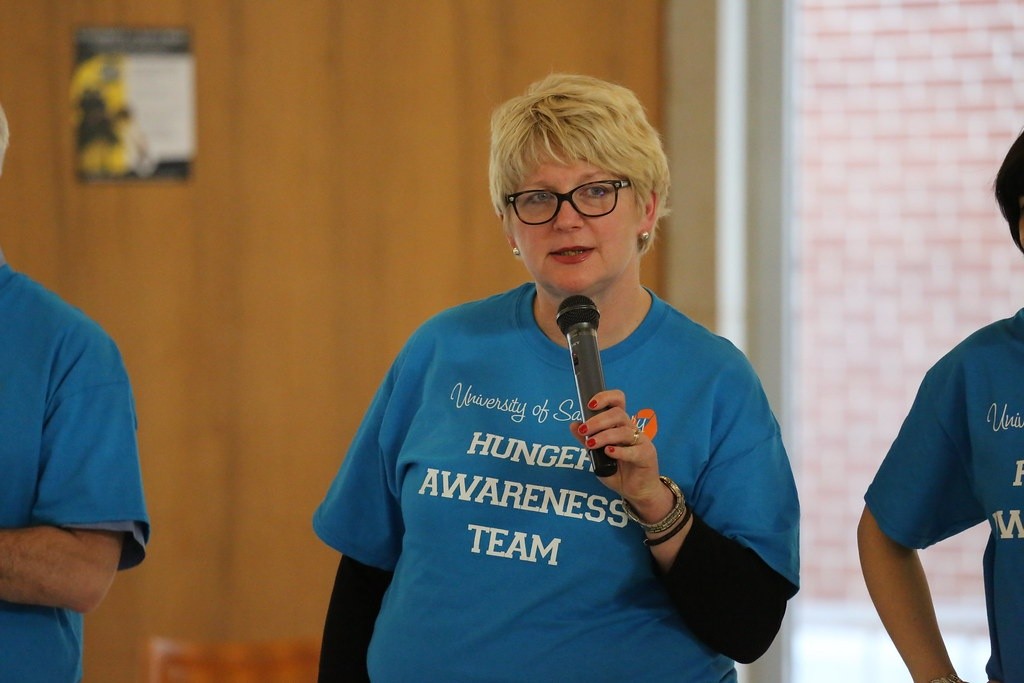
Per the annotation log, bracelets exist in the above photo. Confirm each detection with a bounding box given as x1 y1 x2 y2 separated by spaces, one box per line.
930 675 958 683
639 501 692 546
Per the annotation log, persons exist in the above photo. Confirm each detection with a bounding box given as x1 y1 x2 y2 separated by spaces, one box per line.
313 75 800 683
859 134 1024 683
0 109 149 683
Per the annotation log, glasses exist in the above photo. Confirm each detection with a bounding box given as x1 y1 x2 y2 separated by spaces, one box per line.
503 179 631 225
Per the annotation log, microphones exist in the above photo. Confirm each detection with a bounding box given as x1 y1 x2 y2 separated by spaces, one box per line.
556 294 618 478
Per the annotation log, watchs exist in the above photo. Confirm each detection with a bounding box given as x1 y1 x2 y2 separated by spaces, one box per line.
623 476 685 532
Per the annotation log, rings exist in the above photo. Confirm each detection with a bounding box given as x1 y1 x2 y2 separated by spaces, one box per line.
631 430 638 444
